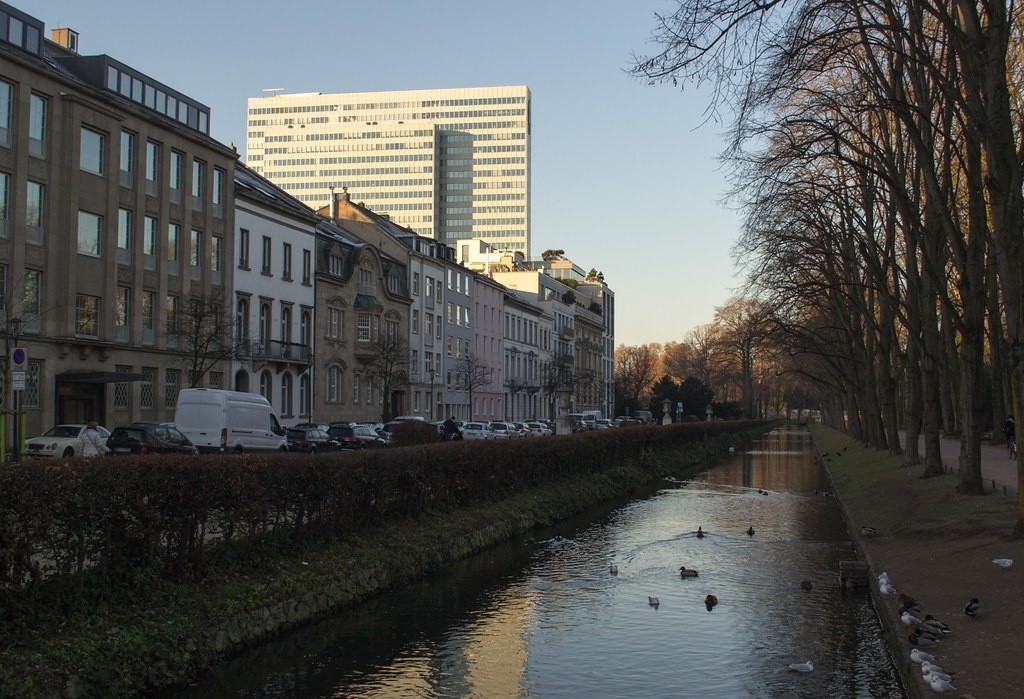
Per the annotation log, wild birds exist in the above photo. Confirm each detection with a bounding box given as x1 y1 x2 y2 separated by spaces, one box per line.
678 565 699 577
814 488 818 492
759 489 769 496
964 597 983 618
801 579 811 588
648 596 659 606
697 526 703 533
706 595 719 606
860 525 877 536
878 571 958 697
747 526 756 535
789 660 814 673
608 565 618 574
814 444 870 466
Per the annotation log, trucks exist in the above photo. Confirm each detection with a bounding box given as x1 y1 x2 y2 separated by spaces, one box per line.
582 411 602 428
570 414 586 431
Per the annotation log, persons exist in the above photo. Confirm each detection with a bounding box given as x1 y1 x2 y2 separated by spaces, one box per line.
443 416 459 441
80 421 112 459
1002 415 1016 448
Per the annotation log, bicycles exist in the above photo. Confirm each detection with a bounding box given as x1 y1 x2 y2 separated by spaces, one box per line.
1003 429 1017 460
440 430 463 441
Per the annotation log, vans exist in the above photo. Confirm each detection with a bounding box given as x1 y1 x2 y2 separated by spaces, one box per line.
381 416 424 447
174 387 288 453
597 419 612 429
635 411 653 422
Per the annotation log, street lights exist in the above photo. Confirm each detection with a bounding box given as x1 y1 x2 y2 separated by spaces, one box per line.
10 317 23 461
429 368 435 421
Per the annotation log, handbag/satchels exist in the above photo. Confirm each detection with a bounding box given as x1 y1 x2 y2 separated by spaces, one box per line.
95 453 105 459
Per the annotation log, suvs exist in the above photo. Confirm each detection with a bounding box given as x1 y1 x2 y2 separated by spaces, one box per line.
105 421 198 455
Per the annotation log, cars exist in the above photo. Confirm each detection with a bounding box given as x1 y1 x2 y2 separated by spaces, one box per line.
615 416 630 427
513 422 531 438
461 423 495 441
297 422 382 432
286 424 384 454
25 423 111 461
489 421 520 440
524 419 553 436
